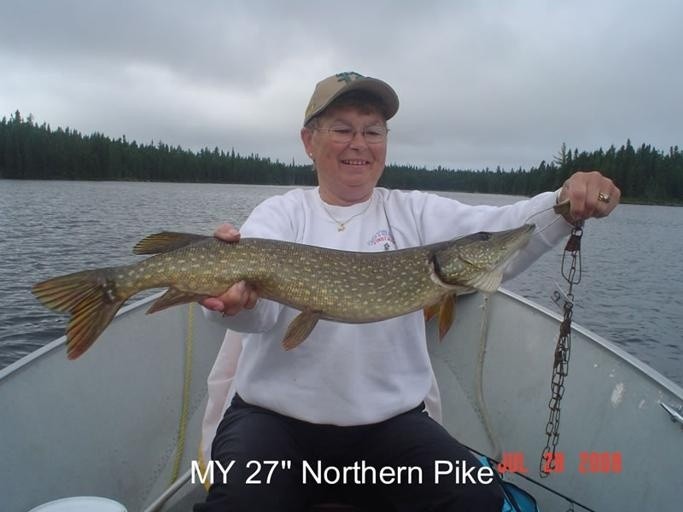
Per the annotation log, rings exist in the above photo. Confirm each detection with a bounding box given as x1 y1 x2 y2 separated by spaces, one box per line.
598 192 611 203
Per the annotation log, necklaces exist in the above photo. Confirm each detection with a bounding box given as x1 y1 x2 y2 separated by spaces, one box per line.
322 203 370 233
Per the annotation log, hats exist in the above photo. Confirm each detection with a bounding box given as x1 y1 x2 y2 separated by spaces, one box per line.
304 71 399 126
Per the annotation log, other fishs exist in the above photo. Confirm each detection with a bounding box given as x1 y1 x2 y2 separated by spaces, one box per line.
28 223 534 359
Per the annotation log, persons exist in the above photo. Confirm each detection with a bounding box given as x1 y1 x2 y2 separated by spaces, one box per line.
195 71 624 511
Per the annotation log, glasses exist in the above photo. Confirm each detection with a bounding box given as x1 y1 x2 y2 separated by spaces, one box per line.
310 120 391 142
